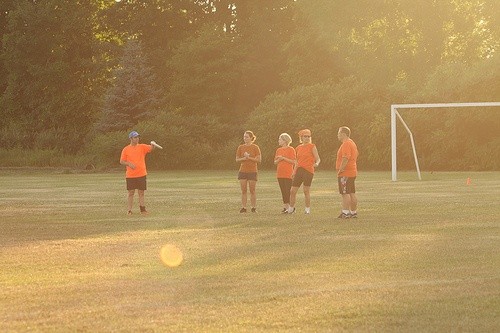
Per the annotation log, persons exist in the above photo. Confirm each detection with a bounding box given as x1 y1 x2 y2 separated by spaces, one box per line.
120 131 158 215
288 129 320 215
273 133 296 214
336 127 359 219
236 131 261 214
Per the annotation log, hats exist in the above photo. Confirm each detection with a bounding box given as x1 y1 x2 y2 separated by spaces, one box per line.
128 131 141 138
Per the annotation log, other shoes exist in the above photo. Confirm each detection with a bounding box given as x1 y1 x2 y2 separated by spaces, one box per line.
239 207 247 213
338 213 357 218
139 205 150 214
251 207 256 213
281 208 296 215
304 208 310 215
127 210 132 215
